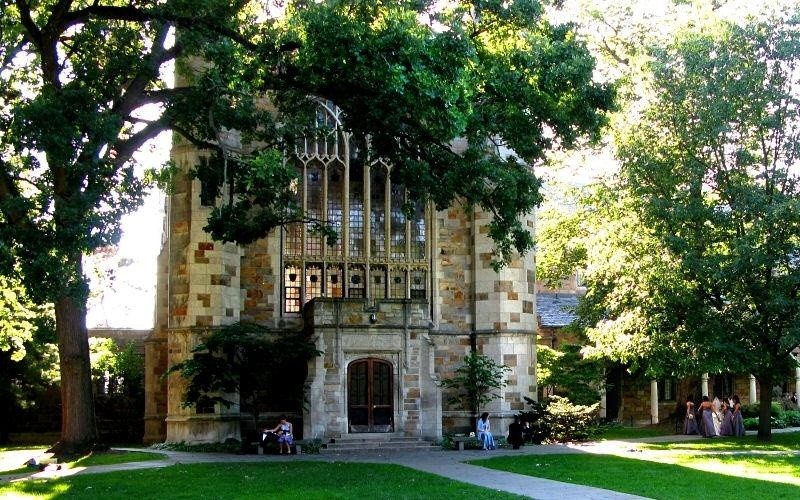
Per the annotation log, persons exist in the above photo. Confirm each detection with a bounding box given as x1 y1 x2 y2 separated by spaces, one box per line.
236 430 286 456
507 417 530 451
265 414 293 455
477 411 495 451
684 394 746 437
791 391 797 403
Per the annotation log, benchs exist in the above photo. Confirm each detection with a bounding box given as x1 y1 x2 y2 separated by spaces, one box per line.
449 433 505 451
252 439 308 454
